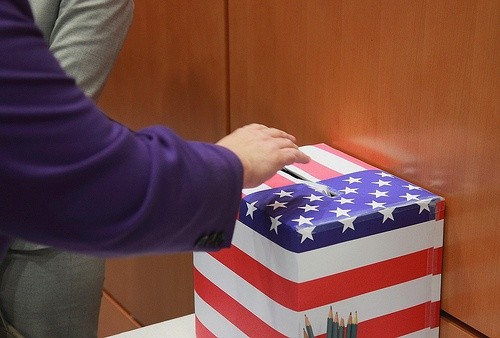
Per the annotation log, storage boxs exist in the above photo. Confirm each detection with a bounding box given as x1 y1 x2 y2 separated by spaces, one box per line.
192 142 445 338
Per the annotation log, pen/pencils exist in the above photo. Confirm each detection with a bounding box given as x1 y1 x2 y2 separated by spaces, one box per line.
326 306 358 338
303 314 315 338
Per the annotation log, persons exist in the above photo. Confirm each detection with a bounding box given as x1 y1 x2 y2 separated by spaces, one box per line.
0 0 312 267
0 0 136 338
